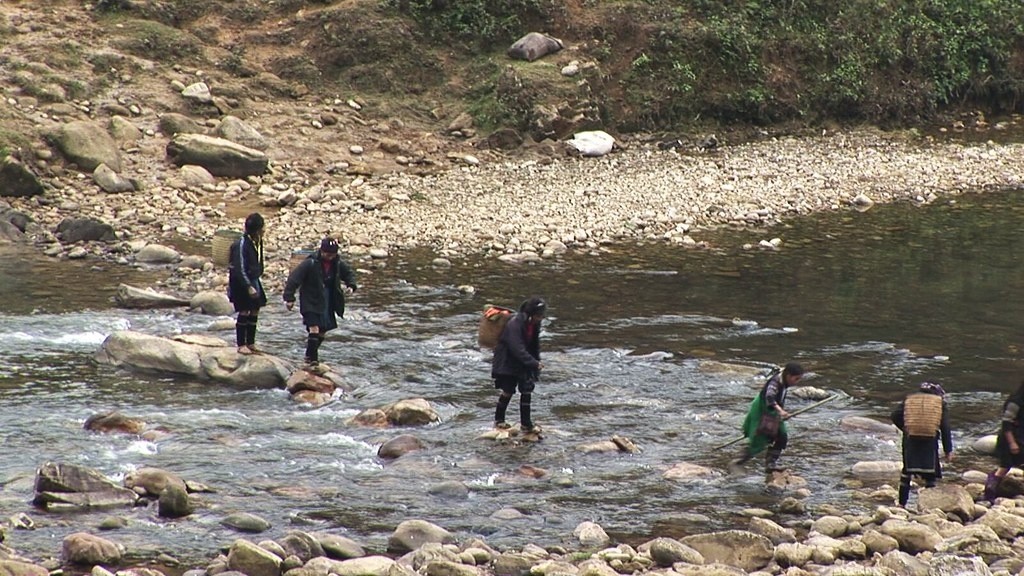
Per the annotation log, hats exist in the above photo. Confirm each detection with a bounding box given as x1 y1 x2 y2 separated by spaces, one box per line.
322 237 339 253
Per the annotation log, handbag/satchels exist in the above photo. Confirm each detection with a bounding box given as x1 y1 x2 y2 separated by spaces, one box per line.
757 415 779 437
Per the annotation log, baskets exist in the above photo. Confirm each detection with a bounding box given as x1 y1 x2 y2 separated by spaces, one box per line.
478 304 516 349
902 393 942 436
288 250 315 276
211 230 243 266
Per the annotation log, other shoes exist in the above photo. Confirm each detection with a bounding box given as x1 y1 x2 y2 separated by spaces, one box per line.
247 344 262 354
237 345 252 354
310 360 319 366
495 421 511 430
304 356 319 363
521 424 542 433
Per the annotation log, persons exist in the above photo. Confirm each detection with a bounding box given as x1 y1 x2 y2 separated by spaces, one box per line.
227 212 268 356
736 363 805 474
282 236 357 367
890 379 953 510
491 298 546 435
984 384 1024 496
